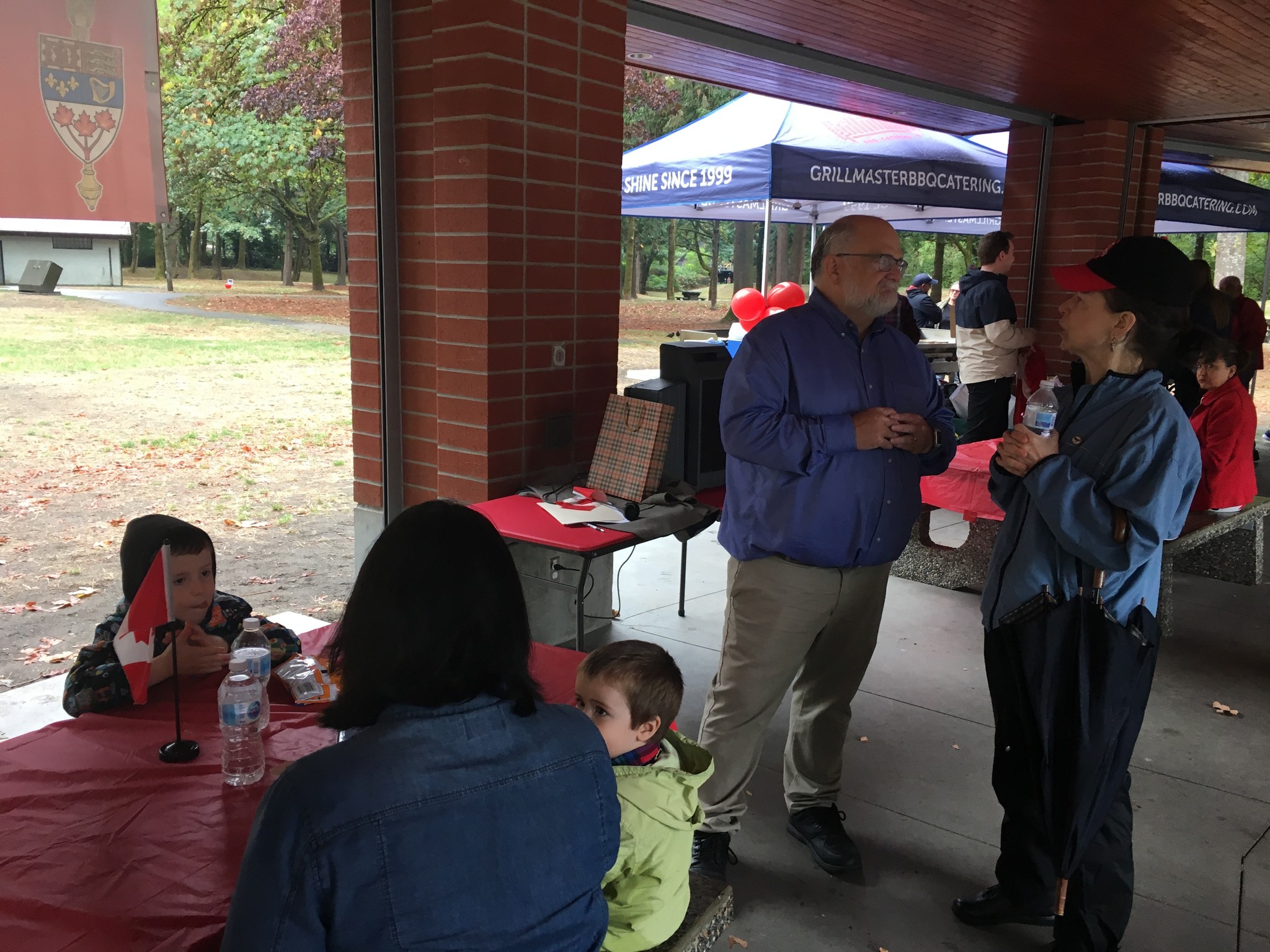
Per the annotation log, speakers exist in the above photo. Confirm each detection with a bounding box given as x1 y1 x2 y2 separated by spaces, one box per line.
659 341 733 491
624 378 687 493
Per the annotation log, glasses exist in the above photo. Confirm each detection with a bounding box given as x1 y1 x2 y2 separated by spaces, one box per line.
836 253 909 277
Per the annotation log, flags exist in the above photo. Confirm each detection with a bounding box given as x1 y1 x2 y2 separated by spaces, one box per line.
112 544 175 704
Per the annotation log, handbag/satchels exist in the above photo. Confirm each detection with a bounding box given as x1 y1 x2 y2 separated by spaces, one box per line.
586 393 676 504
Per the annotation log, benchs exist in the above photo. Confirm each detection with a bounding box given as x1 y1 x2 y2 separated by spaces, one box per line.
650 871 733 952
697 298 706 301
675 297 688 300
1156 496 1270 641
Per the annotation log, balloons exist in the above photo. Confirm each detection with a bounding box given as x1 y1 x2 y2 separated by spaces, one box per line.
763 307 785 318
731 288 766 322
739 314 762 332
765 282 805 310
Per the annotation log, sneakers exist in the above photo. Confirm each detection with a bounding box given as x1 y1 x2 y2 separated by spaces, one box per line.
787 803 861 873
689 829 738 882
951 883 1055 925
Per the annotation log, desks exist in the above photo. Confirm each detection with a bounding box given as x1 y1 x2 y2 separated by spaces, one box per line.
466 486 726 653
917 338 959 383
890 438 1006 590
0 622 677 952
680 291 701 300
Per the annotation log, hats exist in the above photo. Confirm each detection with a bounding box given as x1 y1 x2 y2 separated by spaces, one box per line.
1050 235 1199 309
913 273 939 286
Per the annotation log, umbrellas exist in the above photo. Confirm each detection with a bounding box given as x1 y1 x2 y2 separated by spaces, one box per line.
987 509 1161 917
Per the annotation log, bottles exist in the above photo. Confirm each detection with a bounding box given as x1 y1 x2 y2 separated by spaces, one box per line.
1023 379 1060 438
229 617 270 730
217 659 263 786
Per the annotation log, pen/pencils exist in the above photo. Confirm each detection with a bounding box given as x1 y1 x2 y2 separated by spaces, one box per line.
582 522 604 531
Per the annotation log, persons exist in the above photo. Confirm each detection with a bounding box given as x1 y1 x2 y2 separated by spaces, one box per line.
1219 276 1267 461
1172 259 1232 418
63 515 302 718
906 272 942 329
570 640 715 952
882 292 921 345
935 281 960 386
220 499 621 952
951 235 1204 952
954 231 1038 444
687 215 959 874
1181 347 1258 513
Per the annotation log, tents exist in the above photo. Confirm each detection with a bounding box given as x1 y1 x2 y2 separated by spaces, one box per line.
828 129 1270 401
621 90 1006 306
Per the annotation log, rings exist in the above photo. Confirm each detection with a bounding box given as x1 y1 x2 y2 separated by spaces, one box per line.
914 436 917 443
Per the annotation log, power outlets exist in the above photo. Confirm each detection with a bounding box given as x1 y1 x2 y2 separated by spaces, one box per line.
549 556 560 583
